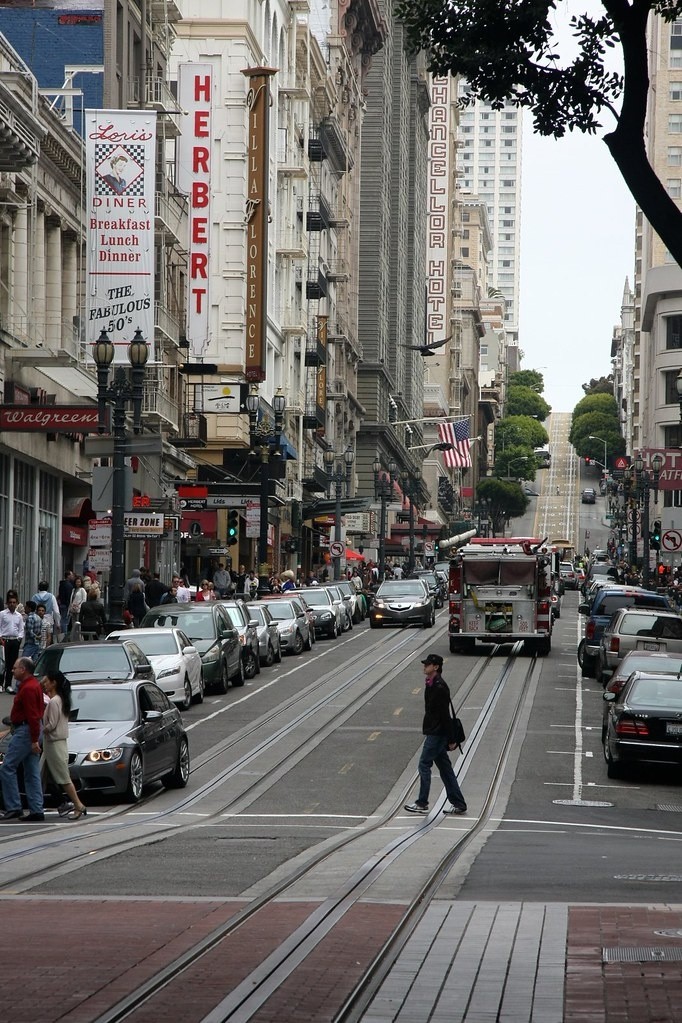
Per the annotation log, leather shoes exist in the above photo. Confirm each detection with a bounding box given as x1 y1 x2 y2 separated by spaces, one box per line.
0 809 24 821
18 813 45 822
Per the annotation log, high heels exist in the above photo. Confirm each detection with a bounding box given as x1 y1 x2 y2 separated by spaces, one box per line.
68 807 86 820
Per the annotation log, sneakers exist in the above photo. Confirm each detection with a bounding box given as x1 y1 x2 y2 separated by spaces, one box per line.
404 802 428 815
0 686 3 693
442 805 467 815
58 802 75 816
6 686 14 693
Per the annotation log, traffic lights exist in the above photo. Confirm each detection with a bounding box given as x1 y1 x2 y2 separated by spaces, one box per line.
653 520 665 576
585 457 589 468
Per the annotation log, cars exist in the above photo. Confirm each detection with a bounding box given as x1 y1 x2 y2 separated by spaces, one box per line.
105 631 205 710
603 671 682 778
560 561 580 589
214 579 369 679
602 649 682 742
581 549 630 604
141 602 247 695
407 562 450 609
0 677 191 806
581 478 608 506
367 577 435 627
32 642 156 693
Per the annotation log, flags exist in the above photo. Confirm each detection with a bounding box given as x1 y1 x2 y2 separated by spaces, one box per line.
438 418 472 468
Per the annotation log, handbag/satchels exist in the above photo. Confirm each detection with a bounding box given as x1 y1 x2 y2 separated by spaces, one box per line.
443 718 465 754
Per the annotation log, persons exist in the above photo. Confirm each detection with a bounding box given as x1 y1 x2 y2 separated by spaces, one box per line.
0 558 107 694
125 563 259 628
268 569 320 594
0 656 88 822
580 547 590 571
607 539 613 560
344 553 424 590
556 486 560 496
614 552 682 613
403 653 469 815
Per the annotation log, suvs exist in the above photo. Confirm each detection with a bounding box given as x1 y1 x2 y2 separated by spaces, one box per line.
600 610 682 682
577 589 675 678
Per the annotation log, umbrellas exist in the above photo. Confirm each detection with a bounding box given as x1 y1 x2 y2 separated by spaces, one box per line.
326 548 365 564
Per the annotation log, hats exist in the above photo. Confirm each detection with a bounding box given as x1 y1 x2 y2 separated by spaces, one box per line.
421 654 443 666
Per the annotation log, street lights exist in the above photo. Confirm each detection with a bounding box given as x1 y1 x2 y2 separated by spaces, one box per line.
633 454 665 590
95 330 421 632
589 436 607 478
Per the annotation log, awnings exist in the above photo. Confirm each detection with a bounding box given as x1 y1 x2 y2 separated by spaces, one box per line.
379 471 418 516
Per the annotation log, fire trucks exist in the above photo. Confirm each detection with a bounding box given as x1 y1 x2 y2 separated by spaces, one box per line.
448 536 560 657
